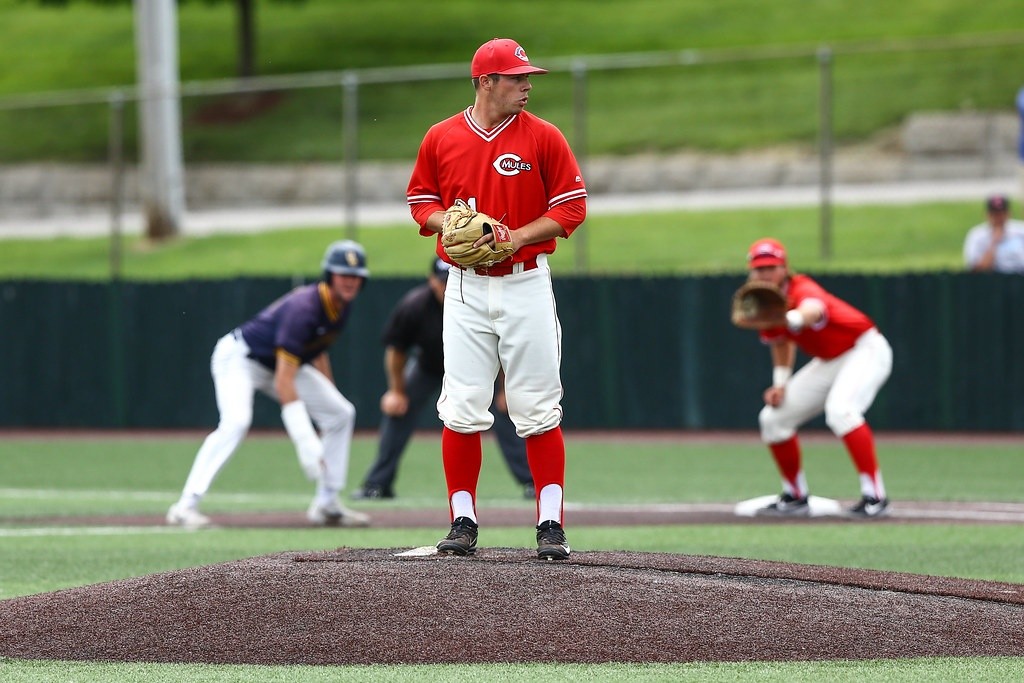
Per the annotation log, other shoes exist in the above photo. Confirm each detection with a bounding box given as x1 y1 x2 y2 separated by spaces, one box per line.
350 488 395 502
523 483 536 500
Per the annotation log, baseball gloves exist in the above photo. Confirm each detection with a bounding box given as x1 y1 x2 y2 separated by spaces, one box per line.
440 200 513 266
732 281 790 330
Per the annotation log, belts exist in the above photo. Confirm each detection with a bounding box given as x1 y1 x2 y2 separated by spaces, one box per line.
456 257 538 277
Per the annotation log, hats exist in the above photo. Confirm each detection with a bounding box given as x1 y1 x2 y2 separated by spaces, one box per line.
432 256 452 285
988 197 1009 213
748 238 788 270
471 38 548 78
323 235 371 279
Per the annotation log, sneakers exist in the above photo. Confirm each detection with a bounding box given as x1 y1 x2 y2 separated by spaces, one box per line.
536 520 571 561
435 516 479 557
754 491 810 519
164 503 211 529
309 505 371 528
840 494 895 521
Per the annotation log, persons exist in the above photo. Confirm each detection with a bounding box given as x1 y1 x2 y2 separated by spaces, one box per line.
731 237 894 521
346 252 536 501
164 240 371 527
962 194 1024 275
406 37 588 560
